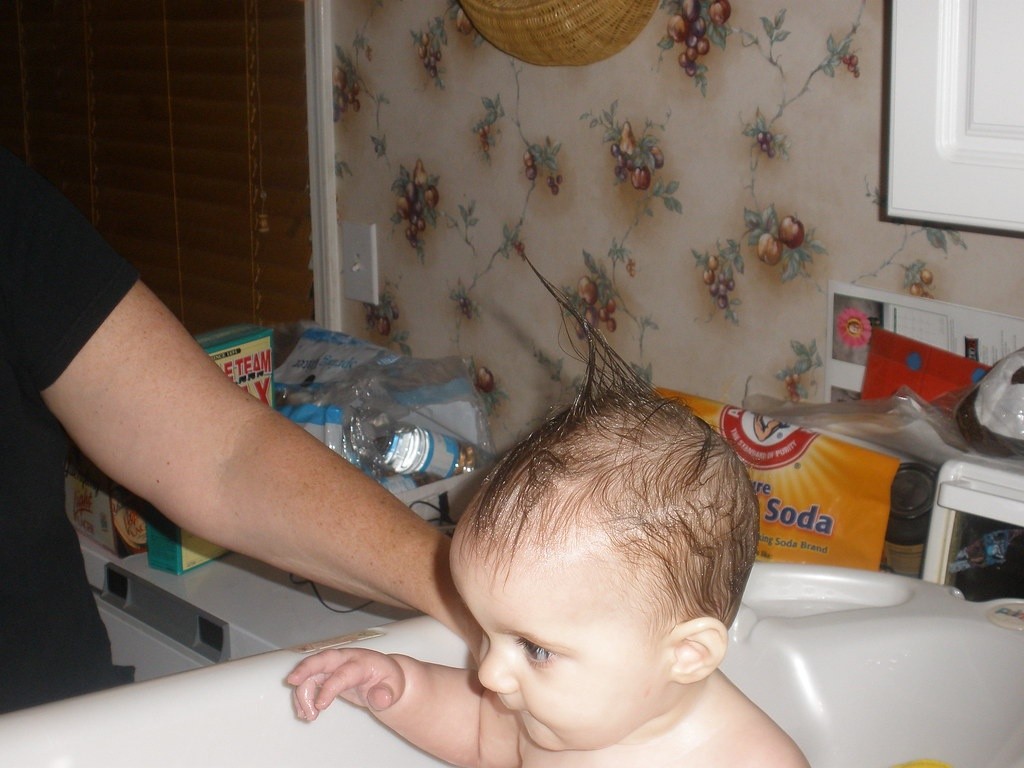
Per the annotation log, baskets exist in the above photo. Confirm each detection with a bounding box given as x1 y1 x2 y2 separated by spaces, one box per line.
460 0 661 66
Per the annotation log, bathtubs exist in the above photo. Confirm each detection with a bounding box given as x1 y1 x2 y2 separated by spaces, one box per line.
0 558 1022 767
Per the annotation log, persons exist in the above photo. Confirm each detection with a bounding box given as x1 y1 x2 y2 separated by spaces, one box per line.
286 389 812 768
0 144 484 715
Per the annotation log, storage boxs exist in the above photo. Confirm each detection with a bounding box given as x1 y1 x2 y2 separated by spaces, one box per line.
60 455 146 561
148 324 276 576
